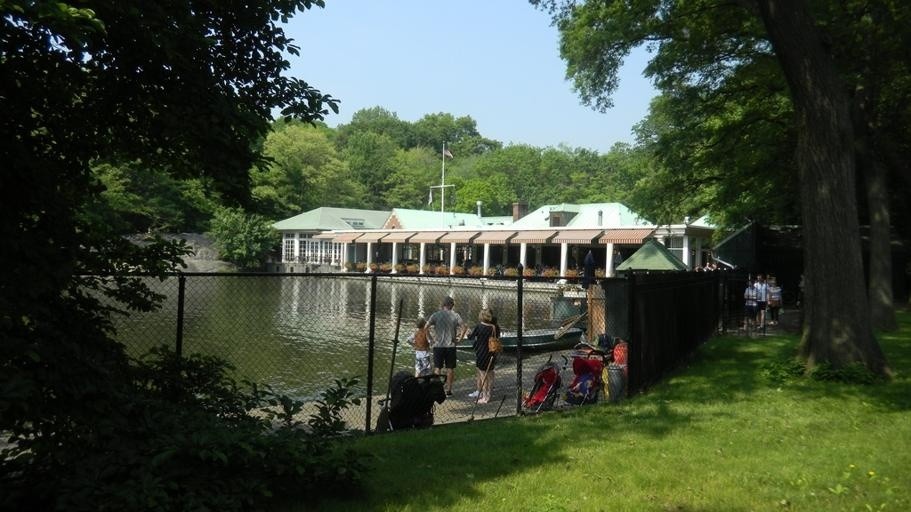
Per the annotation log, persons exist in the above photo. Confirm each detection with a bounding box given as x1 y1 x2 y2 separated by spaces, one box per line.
464 307 502 399
406 316 432 384
424 295 468 399
465 308 499 405
742 271 784 334
692 260 720 273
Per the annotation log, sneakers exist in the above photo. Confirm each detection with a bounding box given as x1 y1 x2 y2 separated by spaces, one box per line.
469 389 490 404
446 390 454 398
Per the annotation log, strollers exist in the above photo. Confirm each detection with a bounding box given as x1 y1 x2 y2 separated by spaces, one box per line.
521 353 568 413
375 371 448 432
563 350 605 407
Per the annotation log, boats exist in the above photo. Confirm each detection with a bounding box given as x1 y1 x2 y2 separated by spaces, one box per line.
406 327 584 365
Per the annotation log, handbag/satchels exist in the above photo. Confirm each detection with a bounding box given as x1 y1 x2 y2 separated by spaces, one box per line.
770 299 780 307
488 337 503 353
